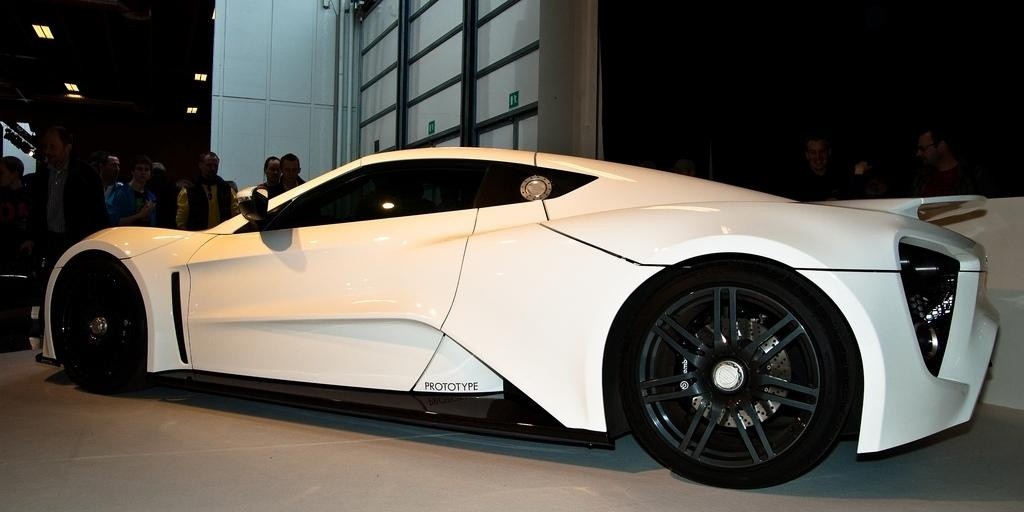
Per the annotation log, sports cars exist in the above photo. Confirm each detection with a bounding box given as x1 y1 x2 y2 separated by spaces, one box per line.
34 144 999 489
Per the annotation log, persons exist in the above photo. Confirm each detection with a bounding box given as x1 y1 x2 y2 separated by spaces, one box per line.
0 129 305 367
783 132 853 201
910 128 990 197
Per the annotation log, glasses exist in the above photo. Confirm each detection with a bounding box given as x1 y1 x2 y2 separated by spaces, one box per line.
207 186 212 200
